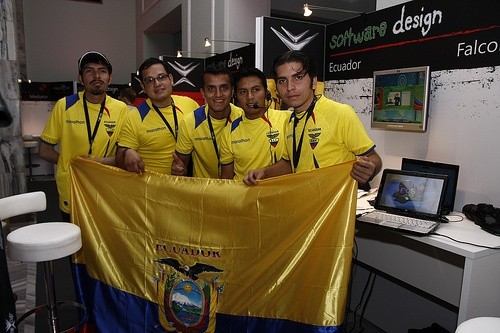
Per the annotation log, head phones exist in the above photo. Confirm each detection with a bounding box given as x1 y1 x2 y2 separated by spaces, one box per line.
233 90 271 103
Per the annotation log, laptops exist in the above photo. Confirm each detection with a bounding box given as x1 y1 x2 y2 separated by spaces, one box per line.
357 169 448 236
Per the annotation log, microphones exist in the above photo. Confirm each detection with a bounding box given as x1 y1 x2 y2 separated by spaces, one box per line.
253 99 272 109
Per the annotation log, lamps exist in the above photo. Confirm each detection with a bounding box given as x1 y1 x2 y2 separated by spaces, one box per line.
303 4 366 17
177 50 218 57
205 37 253 47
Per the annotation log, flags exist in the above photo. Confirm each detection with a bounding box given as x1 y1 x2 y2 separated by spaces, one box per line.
70 153 357 332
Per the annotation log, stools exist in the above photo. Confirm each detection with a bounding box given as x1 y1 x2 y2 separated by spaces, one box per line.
24 134 41 179
0 191 90 333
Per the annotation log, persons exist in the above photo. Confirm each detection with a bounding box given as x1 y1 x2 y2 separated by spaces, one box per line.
171 64 244 176
38 50 127 223
116 57 201 177
242 50 382 187
120 88 136 105
219 68 293 180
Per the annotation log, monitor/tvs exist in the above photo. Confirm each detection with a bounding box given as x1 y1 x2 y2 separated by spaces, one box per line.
400 158 459 214
371 66 431 132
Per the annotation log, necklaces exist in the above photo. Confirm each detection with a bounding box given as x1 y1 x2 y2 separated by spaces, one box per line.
151 97 178 143
207 107 231 167
292 95 317 173
82 92 106 155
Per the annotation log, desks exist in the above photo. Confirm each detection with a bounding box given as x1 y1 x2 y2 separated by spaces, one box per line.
347 185 500 333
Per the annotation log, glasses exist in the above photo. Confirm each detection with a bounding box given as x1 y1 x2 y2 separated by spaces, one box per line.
143 74 168 84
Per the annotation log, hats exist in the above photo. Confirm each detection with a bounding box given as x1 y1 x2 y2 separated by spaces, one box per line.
78 50 113 74
